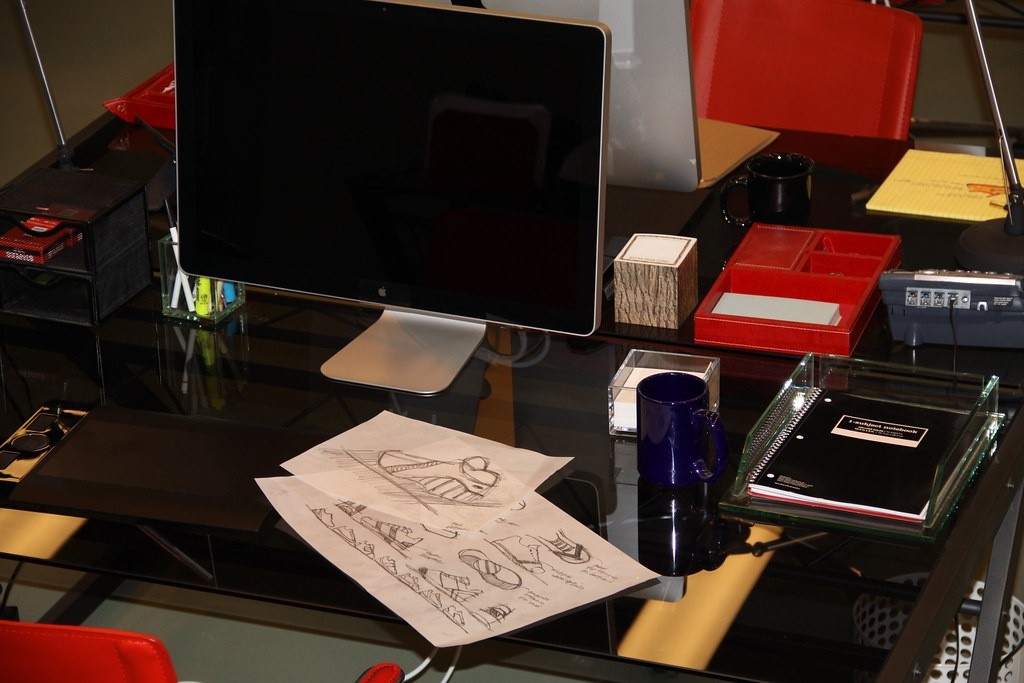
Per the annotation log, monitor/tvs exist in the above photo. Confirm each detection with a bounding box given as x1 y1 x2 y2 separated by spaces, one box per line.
172 0 615 396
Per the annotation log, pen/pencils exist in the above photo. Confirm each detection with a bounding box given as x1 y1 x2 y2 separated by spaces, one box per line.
160 193 238 316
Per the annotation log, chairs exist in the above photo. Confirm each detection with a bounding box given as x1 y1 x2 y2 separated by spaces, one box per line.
689 0 922 140
2 621 407 682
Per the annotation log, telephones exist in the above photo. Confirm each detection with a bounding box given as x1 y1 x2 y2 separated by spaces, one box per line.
877 268 1024 349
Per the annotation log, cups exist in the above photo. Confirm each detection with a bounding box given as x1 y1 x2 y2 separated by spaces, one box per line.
157 229 247 324
720 150 816 228
636 371 729 487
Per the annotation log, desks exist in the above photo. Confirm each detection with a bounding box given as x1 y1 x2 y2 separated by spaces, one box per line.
1 61 1022 682
